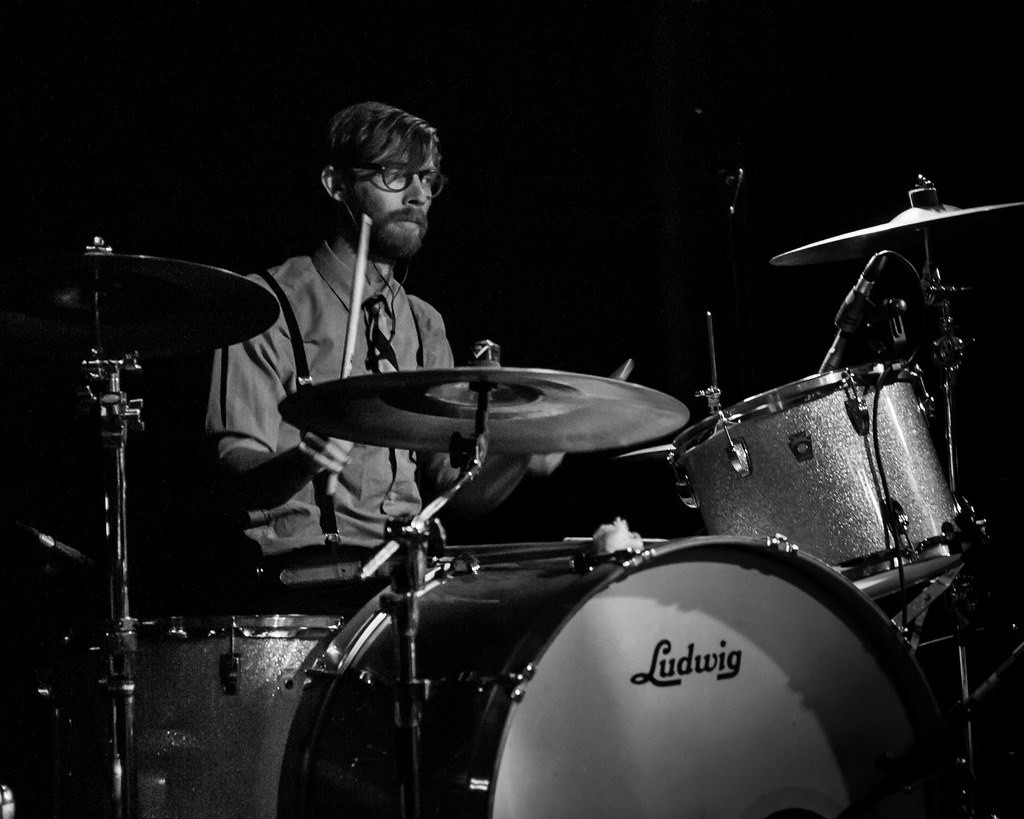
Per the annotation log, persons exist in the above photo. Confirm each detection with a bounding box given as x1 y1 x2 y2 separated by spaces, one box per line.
200 98 568 615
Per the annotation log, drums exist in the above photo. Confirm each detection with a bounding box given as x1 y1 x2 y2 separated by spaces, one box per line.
113 610 356 819
276 530 955 819
673 361 971 602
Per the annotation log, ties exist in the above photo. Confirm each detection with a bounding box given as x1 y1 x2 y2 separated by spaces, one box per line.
366 294 398 374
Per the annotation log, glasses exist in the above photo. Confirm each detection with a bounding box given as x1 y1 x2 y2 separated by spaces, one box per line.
340 160 448 199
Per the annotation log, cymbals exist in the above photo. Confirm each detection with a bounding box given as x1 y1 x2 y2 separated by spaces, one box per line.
0 247 281 356
769 201 1024 267
279 363 691 455
